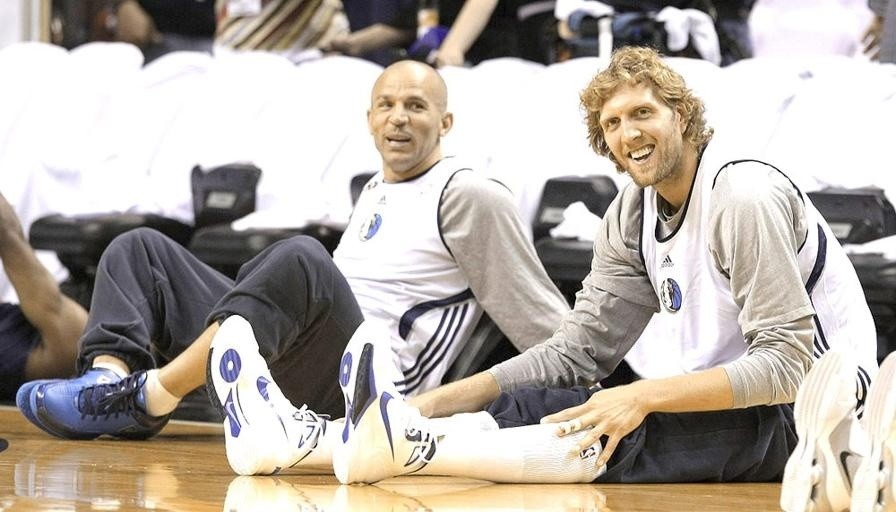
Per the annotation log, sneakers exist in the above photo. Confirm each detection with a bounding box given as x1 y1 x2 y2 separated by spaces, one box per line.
853 351 896 512
775 344 871 512
12 444 151 506
15 366 119 441
31 375 171 442
331 315 440 486
219 476 404 512
205 315 323 475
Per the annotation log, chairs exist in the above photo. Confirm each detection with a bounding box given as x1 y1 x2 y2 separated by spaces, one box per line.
0 39 896 329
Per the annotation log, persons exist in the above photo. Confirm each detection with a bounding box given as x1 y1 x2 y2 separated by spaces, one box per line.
12 57 578 444
203 47 878 484
38 0 895 66
0 191 90 408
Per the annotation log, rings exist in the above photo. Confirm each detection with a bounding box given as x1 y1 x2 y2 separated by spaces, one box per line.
569 417 581 432
559 422 571 434
